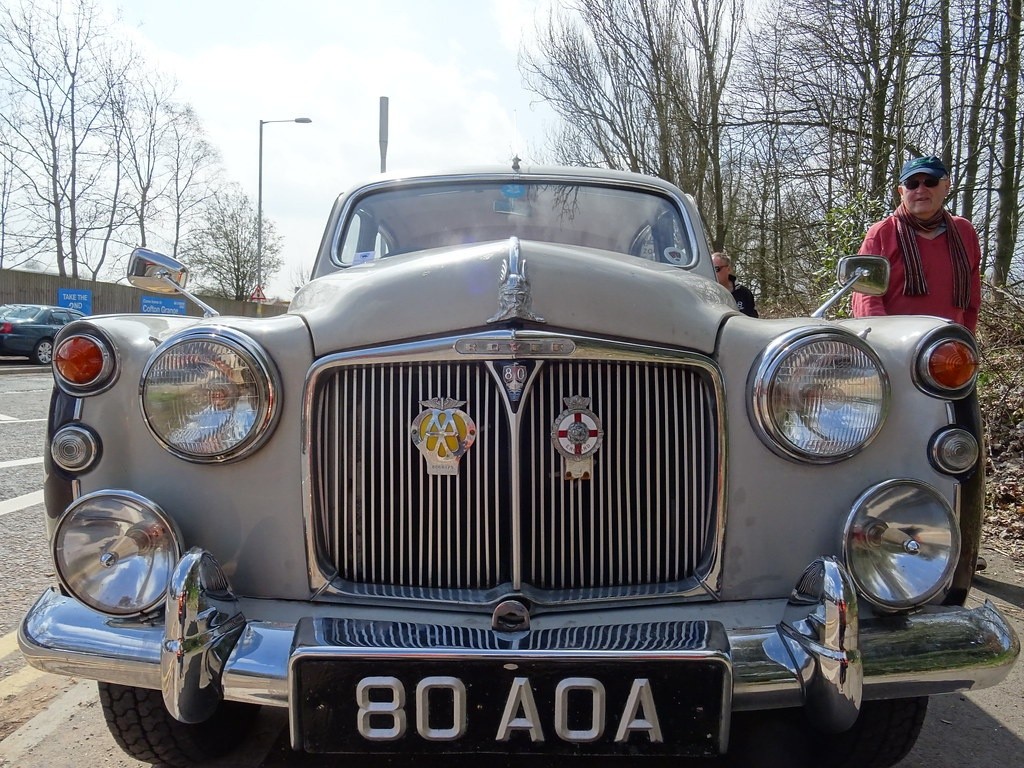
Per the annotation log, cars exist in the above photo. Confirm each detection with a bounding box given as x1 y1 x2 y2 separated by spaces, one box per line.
0 304 87 366
16 153 1020 768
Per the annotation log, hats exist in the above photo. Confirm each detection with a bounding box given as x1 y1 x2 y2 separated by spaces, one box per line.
899 156 948 182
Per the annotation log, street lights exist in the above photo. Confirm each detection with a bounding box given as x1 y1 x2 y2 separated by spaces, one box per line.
248 117 312 318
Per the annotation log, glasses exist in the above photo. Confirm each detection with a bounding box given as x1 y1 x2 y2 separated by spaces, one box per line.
714 266 730 273
901 176 945 190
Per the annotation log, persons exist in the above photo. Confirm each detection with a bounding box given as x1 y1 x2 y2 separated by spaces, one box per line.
852 156 981 335
712 252 759 318
